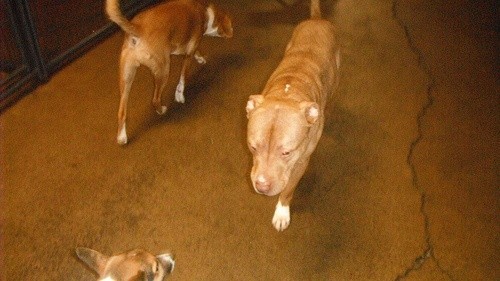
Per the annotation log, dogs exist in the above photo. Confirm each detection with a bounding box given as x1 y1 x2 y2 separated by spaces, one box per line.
105 0 234 145
246 0 340 231
73 246 176 281
